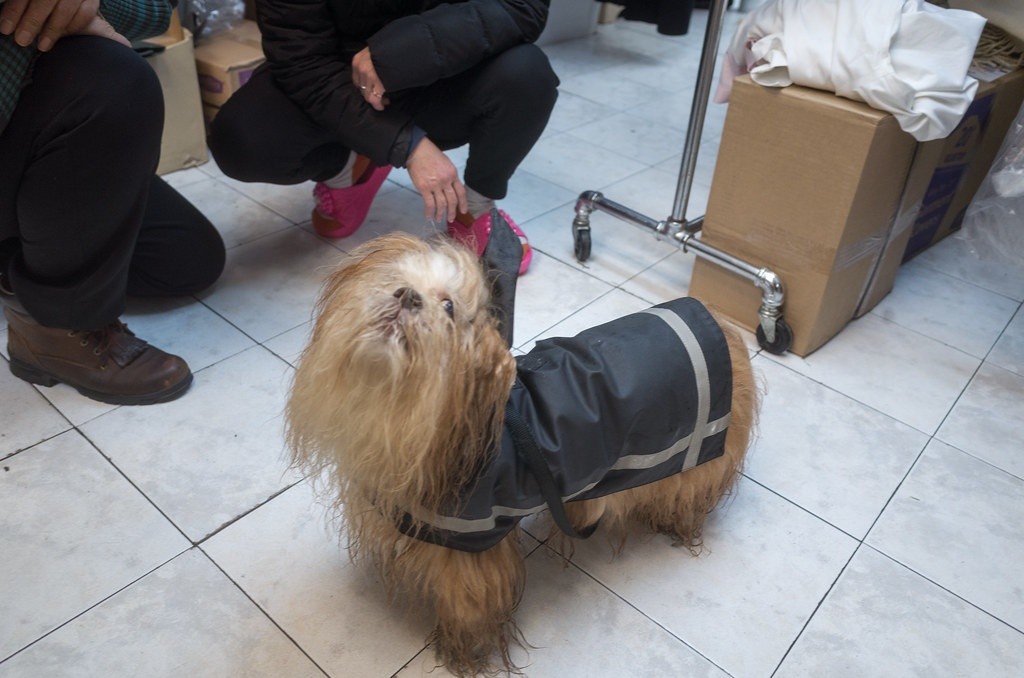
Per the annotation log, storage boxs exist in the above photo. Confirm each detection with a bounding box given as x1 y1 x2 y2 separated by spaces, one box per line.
193 18 265 124
900 22 1024 268
687 73 943 357
143 27 209 175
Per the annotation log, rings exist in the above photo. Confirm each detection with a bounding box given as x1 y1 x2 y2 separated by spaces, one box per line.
372 90 382 99
360 86 367 90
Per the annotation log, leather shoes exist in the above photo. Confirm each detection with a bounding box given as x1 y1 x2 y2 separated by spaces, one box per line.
4 304 193 406
0 254 15 295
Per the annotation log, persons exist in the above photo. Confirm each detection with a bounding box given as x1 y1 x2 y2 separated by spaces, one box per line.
206 0 560 276
0 0 227 406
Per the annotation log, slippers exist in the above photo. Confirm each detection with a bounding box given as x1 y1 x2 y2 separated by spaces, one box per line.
447 210 531 275
310 164 392 239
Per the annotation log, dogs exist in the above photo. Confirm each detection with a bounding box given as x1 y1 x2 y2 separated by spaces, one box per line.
283 226 761 678
